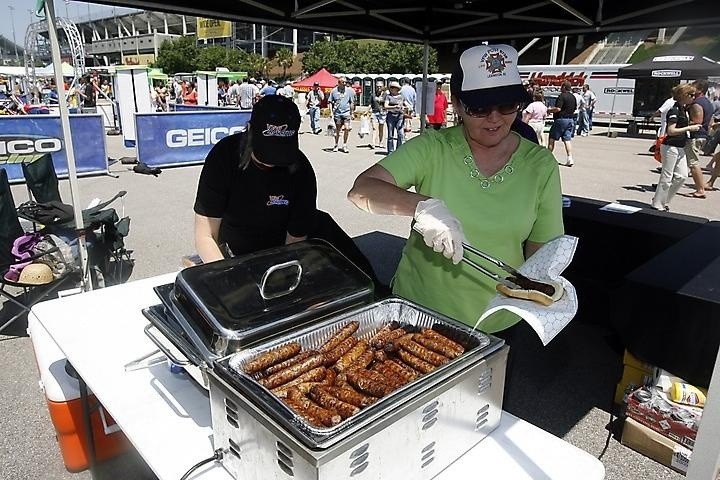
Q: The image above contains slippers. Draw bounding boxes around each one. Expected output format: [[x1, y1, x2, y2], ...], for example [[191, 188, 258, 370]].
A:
[[685, 192, 708, 198]]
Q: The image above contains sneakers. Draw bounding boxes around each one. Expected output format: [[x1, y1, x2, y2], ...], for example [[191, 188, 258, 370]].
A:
[[368, 139, 385, 149], [563, 160, 574, 167], [314, 128, 322, 135], [332, 144, 350, 152]]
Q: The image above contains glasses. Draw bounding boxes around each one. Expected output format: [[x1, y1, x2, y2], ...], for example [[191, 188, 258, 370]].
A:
[[689, 93, 696, 97], [456, 100, 523, 118]]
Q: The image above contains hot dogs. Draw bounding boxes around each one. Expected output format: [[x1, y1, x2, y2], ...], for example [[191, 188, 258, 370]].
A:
[[497, 275, 564, 307]]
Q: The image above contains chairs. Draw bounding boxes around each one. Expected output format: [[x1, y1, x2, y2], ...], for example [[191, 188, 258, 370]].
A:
[[0, 152, 131, 332]]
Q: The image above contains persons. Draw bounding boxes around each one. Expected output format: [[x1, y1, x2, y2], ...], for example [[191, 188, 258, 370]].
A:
[[649, 79, 720, 213], [518, 79, 597, 165], [347, 43, 565, 408], [194, 93, 317, 264]]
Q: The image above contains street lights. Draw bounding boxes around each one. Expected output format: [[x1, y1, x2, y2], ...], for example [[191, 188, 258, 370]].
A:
[[8, 5, 20, 63], [65, 0, 70, 18], [27, 8, 35, 24]]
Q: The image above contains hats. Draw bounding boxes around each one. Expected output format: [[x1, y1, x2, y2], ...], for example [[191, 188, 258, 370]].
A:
[[389, 81, 401, 90], [250, 94, 302, 166], [313, 81, 319, 86], [285, 80, 291, 85], [450, 43, 531, 104]]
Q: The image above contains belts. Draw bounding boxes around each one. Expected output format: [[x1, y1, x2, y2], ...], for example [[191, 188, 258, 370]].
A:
[[555, 115, 574, 119]]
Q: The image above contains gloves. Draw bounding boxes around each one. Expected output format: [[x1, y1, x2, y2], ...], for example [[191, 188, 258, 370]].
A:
[[413, 198, 471, 266]]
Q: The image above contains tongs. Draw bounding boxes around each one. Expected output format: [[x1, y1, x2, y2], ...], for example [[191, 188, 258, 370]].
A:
[[410, 221, 529, 290]]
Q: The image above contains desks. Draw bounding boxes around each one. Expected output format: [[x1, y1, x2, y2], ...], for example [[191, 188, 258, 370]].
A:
[[31, 271, 607, 480]]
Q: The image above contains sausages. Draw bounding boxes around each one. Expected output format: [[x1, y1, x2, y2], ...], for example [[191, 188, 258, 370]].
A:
[[242, 319, 466, 427]]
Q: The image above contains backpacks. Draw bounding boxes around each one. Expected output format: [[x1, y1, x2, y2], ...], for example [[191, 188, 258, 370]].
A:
[[34, 233, 75, 281]]
